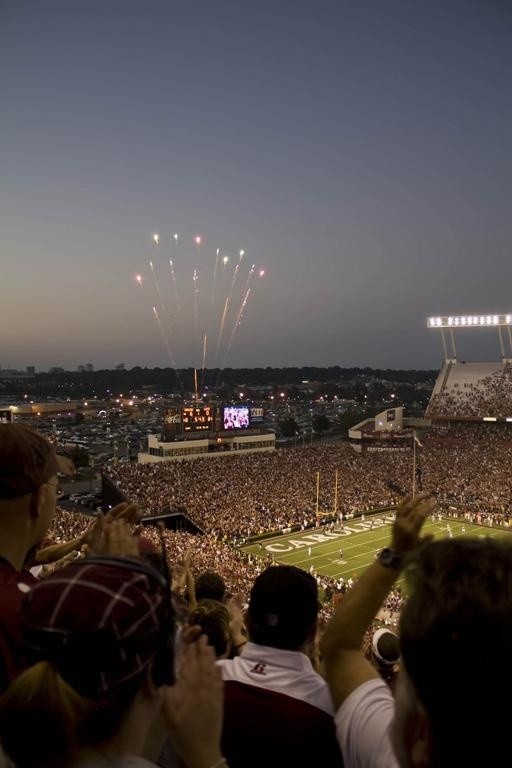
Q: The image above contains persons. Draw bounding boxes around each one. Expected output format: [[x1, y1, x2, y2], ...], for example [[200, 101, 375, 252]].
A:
[[103, 361, 512, 541], [0, 503, 512, 767], [1, 422, 143, 694]]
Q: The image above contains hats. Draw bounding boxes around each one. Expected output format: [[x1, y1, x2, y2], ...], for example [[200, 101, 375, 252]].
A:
[[0, 422, 63, 500], [23, 554, 166, 699], [372, 628, 401, 665], [251, 565, 323, 617]]
